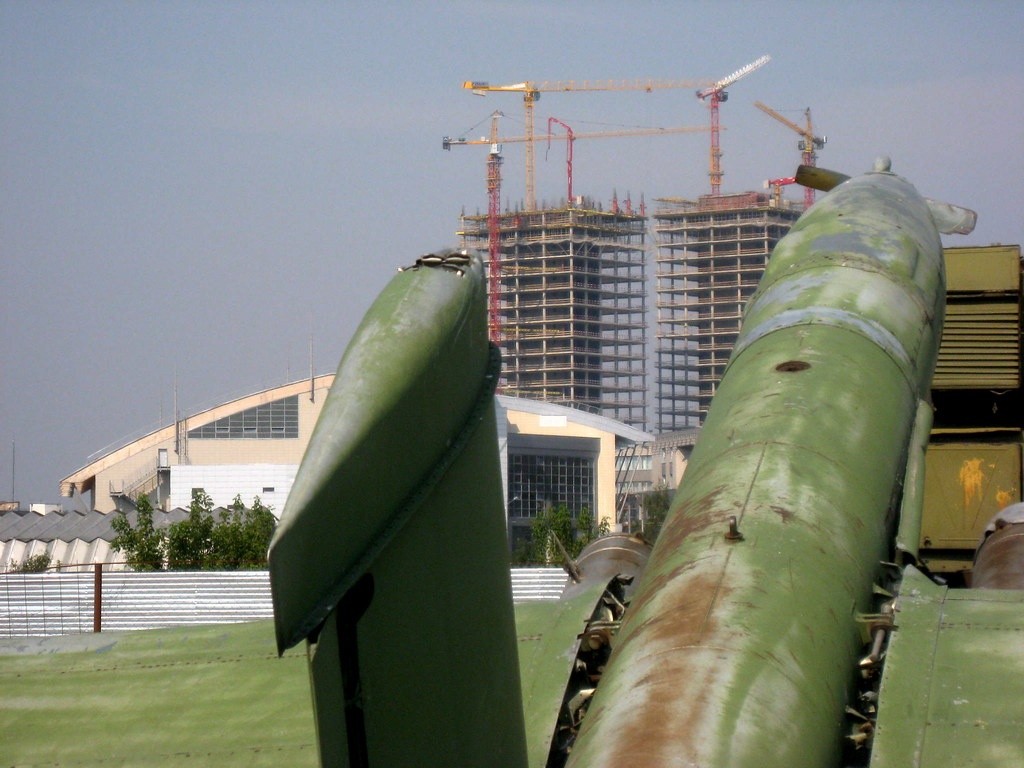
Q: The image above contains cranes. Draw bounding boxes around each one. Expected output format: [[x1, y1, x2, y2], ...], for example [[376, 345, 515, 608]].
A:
[[438, 54, 828, 207]]
